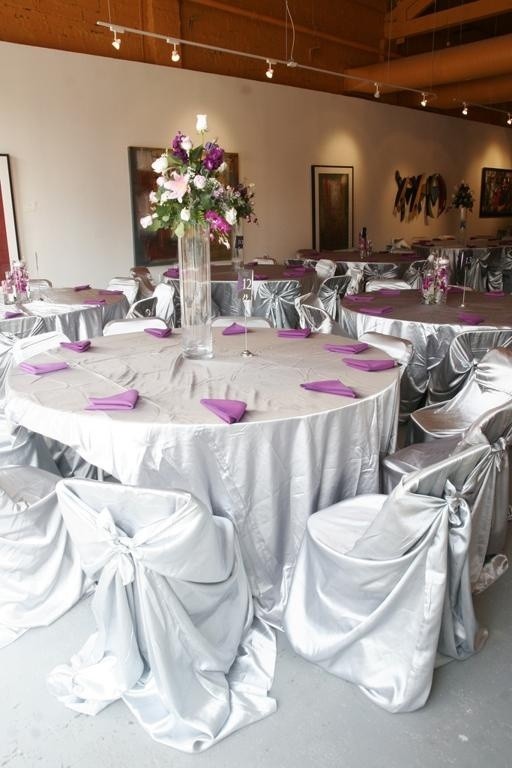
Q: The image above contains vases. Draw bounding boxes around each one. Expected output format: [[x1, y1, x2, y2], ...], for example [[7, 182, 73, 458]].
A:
[[457, 204, 468, 230], [176, 217, 217, 361]]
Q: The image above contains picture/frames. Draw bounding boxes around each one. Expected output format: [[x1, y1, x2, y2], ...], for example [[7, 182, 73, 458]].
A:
[[479, 165, 512, 219], [0, 153, 22, 286], [308, 162, 359, 255], [128, 145, 240, 271]]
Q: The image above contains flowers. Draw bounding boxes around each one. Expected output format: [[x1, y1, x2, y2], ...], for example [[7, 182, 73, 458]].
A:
[[450, 180, 475, 210], [140, 113, 260, 252]]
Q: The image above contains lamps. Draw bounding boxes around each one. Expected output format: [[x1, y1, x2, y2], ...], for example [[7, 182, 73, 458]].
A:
[[96, 20, 512, 124]]
